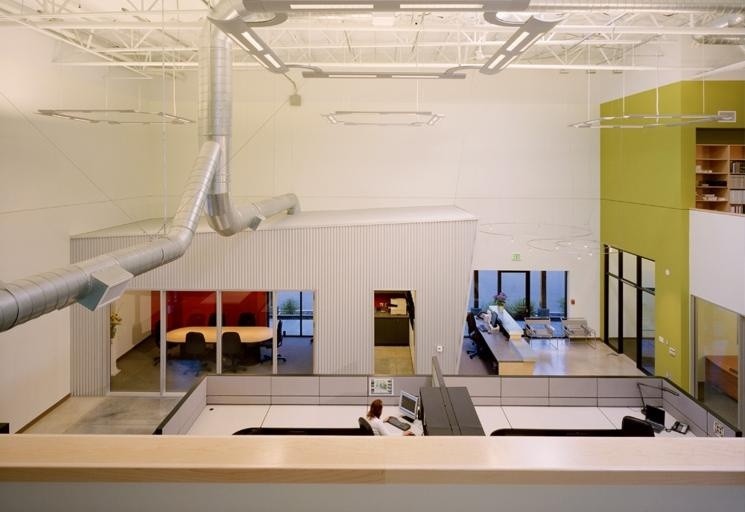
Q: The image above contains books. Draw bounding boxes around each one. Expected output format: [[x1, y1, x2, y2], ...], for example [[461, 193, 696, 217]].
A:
[[730, 160, 745, 213]]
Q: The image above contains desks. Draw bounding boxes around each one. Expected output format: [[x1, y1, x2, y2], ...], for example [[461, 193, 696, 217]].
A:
[[473, 404, 698, 437], [184, 404, 423, 438], [473, 315, 537, 375]]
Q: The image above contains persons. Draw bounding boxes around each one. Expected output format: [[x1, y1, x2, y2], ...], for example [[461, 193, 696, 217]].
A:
[[367, 399, 413, 436]]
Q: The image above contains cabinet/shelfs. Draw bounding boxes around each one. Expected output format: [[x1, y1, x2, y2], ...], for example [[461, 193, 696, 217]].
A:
[[697, 142, 745, 215]]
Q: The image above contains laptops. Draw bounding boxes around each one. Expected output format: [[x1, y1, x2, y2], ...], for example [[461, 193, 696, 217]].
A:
[[645, 405, 665, 433]]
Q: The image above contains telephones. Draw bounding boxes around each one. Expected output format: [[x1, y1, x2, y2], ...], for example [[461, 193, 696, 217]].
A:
[[671, 421, 689, 434]]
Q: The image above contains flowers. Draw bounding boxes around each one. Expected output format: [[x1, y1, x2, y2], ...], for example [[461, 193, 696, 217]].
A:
[[495, 292, 506, 304], [110, 312, 122, 327]]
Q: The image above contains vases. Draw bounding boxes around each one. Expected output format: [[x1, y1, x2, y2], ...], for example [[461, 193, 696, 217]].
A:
[[110, 325, 116, 339]]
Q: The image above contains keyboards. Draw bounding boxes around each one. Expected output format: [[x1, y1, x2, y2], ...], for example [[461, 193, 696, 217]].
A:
[[386, 416, 410, 431], [479, 325, 488, 332]]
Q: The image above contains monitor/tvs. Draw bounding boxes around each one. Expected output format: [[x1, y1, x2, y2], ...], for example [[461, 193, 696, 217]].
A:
[[400, 391, 417, 423], [491, 311, 498, 327]]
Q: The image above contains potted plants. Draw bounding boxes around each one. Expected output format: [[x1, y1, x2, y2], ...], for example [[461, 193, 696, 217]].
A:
[[498, 305, 504, 313]]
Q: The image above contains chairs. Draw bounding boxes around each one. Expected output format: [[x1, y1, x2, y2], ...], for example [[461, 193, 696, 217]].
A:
[[466, 313, 484, 359], [524, 316, 596, 351], [153, 313, 287, 376]]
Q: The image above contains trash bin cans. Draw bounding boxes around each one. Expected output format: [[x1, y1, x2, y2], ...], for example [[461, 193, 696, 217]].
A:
[[698, 381, 705, 400]]
